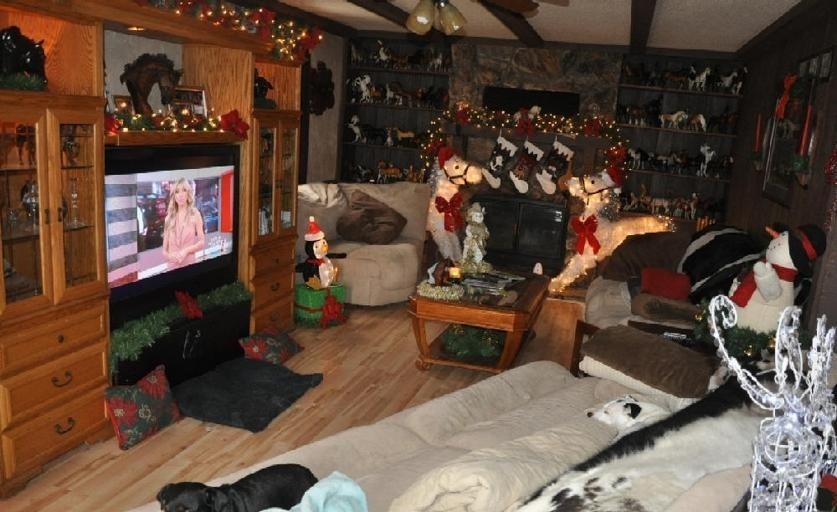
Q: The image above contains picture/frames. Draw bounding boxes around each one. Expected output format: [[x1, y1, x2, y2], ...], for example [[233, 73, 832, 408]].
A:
[[112, 94, 136, 119], [166, 85, 208, 118], [797, 45, 834, 82]]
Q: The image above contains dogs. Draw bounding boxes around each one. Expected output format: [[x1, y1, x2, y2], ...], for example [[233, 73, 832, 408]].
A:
[[156, 463, 318, 512], [587, 394, 671, 444]]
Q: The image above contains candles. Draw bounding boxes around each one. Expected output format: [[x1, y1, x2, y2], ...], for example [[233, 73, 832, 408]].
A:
[[798, 104, 813, 158], [752, 112, 762, 151]]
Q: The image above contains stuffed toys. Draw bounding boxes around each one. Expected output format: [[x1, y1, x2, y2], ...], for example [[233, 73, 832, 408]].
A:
[[725, 219, 826, 337], [460, 200, 489, 263], [294, 213, 348, 327]]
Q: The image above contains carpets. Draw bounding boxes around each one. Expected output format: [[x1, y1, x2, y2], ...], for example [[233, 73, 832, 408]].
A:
[[174, 358, 322, 433]]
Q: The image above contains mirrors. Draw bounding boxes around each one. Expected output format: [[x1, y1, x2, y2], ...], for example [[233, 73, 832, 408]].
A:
[[761, 76, 817, 206]]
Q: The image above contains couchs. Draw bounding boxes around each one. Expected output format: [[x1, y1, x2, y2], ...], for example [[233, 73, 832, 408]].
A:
[[134, 325, 809, 511]]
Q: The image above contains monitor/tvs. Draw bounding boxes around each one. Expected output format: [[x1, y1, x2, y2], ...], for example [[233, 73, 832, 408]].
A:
[[105, 145, 241, 330]]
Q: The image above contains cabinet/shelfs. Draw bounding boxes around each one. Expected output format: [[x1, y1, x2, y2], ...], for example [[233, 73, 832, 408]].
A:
[[1, 91, 109, 487], [470, 194, 566, 276], [614, 83, 743, 224], [245, 109, 296, 341], [345, 61, 449, 155]]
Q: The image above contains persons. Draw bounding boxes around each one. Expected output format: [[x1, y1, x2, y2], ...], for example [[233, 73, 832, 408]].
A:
[[160, 177, 207, 273]]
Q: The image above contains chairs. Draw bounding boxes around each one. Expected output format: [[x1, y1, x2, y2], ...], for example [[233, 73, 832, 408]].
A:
[[298, 183, 433, 306], [570, 226, 809, 376]]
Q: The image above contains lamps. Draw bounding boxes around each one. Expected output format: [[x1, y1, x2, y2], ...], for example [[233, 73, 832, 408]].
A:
[[406, 0, 468, 36]]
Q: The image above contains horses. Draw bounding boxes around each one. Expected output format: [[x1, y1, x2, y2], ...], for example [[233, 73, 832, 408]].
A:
[[513, 105, 541, 124], [121, 53, 184, 114]]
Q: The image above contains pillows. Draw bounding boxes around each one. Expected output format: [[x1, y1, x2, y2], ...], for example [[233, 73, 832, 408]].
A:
[[640, 267, 691, 301], [105, 368, 179, 449], [338, 190, 408, 246], [235, 325, 304, 364]]
